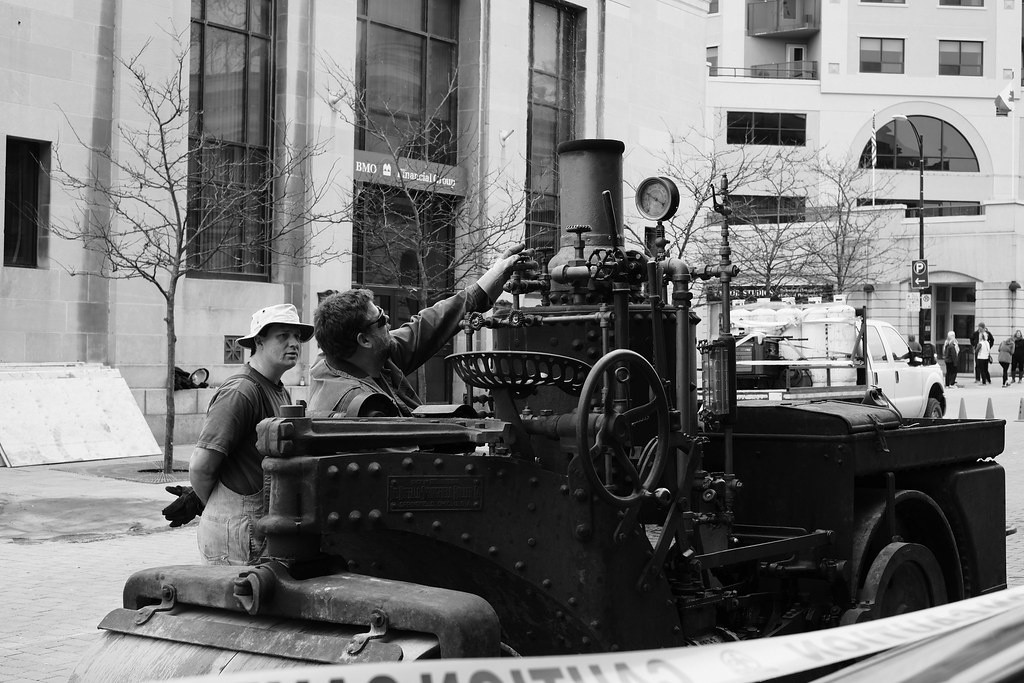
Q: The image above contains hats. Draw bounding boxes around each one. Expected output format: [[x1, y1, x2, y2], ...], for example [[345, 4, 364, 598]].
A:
[[236, 304, 315, 349]]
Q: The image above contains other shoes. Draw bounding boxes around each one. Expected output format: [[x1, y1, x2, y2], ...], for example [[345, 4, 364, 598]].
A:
[[1018, 380, 1022, 383], [945, 384, 958, 389], [974, 380, 992, 386], [1002, 383, 1010, 388], [1011, 380, 1015, 383]]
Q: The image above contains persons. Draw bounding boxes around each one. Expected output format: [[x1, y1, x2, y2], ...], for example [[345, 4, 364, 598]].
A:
[[163, 303, 315, 566], [907, 322, 1024, 389], [304, 244, 526, 422]]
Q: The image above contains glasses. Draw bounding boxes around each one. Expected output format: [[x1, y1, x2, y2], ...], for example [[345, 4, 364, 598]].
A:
[[371, 306, 389, 328]]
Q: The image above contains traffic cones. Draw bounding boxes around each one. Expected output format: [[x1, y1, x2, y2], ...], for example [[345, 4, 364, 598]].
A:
[[985, 397, 995, 419], [958, 397, 968, 419], [1013, 397, 1024, 422]]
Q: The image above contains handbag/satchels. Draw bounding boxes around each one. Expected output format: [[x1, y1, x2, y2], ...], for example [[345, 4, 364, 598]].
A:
[[989, 356, 993, 364]]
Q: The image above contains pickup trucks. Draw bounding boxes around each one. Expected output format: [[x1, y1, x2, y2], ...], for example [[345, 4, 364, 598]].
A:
[[724, 299, 948, 418]]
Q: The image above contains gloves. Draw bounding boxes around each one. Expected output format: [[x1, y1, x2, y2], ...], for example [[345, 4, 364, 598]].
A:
[[162, 485, 205, 527], [476, 243, 539, 302]]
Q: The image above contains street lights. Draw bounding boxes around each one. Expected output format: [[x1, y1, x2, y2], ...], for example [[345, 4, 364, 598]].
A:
[[893, 114, 925, 346]]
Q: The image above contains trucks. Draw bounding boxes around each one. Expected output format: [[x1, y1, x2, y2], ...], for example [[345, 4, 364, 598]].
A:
[[691, 297, 949, 420]]
